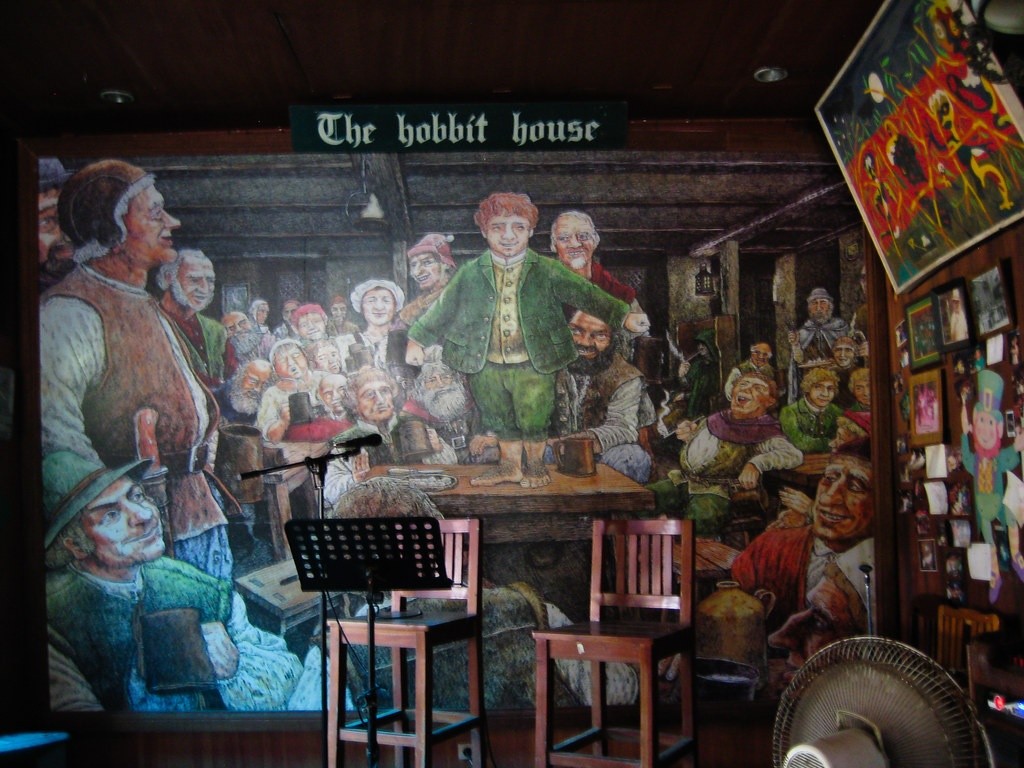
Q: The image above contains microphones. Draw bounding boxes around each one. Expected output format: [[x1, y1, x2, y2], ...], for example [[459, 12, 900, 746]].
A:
[[333, 433, 382, 449]]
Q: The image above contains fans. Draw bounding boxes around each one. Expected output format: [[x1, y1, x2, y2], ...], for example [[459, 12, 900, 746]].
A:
[[770, 635, 993, 768]]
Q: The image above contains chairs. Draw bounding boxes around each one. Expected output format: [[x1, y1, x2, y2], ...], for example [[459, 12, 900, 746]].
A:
[[320, 518, 487, 768], [530, 517, 698, 768]]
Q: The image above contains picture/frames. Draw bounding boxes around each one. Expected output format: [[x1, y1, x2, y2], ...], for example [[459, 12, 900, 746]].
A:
[[902, 288, 949, 373], [931, 276, 977, 356], [967, 255, 1017, 344], [908, 367, 946, 448], [813, 0, 1024, 298]]
